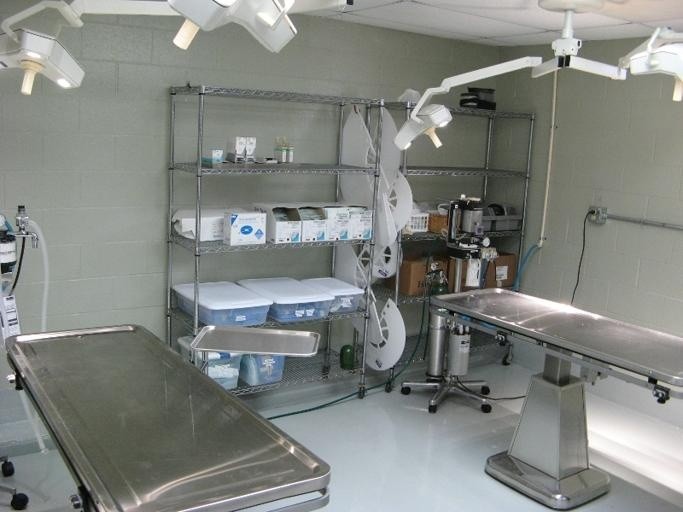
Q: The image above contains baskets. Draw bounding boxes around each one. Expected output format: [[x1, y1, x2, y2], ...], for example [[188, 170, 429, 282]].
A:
[[408, 213, 429, 233], [428, 214, 448, 233]]
